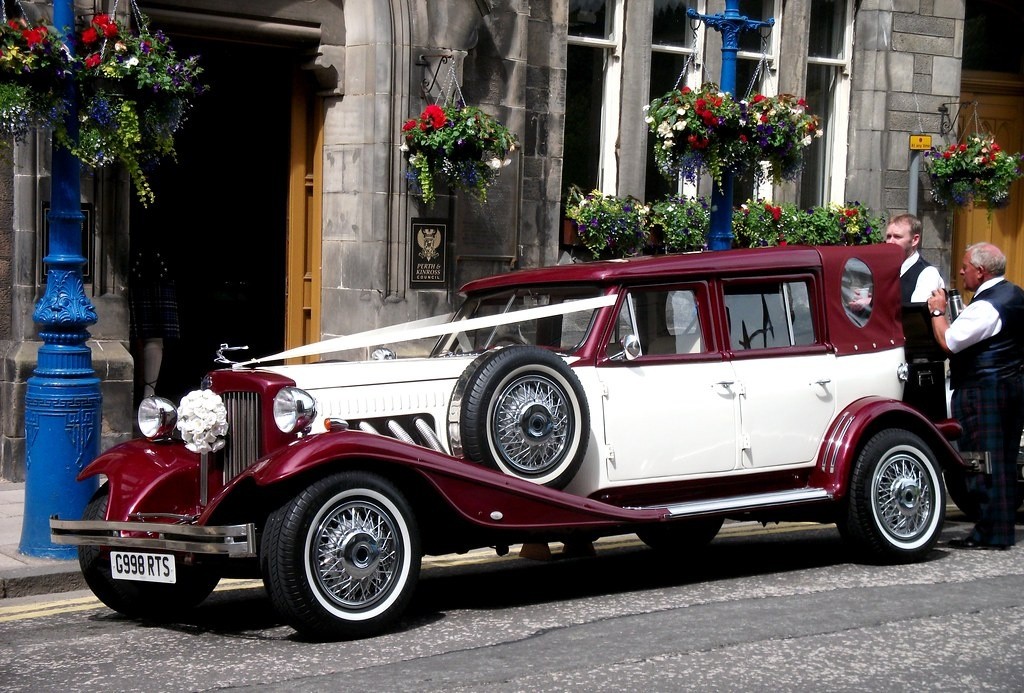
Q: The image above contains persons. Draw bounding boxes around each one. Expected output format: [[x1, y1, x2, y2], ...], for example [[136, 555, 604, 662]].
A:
[[927, 243, 1024, 551], [848, 214, 948, 423]]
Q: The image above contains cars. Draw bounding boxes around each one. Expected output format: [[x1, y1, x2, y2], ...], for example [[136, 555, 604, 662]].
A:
[[48, 244, 993, 640]]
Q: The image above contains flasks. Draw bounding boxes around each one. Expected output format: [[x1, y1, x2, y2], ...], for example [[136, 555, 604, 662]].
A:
[[948, 289, 964, 324]]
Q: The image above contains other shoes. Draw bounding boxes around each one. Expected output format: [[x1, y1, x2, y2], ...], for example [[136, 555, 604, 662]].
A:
[[563, 540, 596, 558], [520, 543, 552, 561]]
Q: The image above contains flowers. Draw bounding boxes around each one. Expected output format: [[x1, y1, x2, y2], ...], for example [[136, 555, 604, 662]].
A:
[[924, 142, 972, 177], [176, 389, 229, 455], [77, 8, 212, 97], [967, 131, 1024, 186], [643, 81, 749, 197], [402, 102, 519, 209], [730, 197, 891, 249], [0, 11, 61, 75], [736, 90, 823, 195], [561, 183, 711, 260]]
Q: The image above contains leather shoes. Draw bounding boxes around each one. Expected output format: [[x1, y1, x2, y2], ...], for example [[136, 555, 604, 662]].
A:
[[947, 535, 1011, 550]]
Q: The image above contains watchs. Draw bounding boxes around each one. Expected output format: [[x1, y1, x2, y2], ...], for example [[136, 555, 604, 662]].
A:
[[931, 309, 945, 318]]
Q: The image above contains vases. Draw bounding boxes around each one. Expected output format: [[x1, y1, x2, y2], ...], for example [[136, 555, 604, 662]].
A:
[[980, 168, 1010, 209], [564, 220, 664, 247], [0, 53, 54, 94], [406, 135, 482, 170], [930, 167, 971, 207], [81, 74, 132, 104]]
